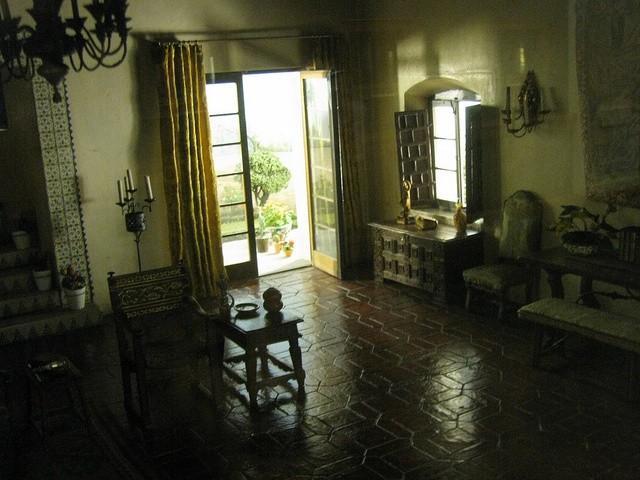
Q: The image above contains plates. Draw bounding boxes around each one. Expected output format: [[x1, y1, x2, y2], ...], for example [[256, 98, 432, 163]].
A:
[[233, 302, 260, 314]]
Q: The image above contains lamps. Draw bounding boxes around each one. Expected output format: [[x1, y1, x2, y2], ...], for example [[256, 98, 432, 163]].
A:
[[1, 1, 131, 106], [500, 69, 557, 140]]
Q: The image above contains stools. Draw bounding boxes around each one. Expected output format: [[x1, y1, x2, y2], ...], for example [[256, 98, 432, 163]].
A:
[[21, 347, 88, 441]]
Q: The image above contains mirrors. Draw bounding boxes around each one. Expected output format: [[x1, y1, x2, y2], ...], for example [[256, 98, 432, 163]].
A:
[[430, 90, 478, 216]]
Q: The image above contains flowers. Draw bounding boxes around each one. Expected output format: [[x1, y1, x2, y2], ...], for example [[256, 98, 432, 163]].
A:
[[58, 264, 89, 290]]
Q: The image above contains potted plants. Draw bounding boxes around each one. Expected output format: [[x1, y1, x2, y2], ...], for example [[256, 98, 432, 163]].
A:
[[31, 265, 55, 295], [272, 231, 284, 255], [10, 222, 34, 252], [283, 241, 296, 257], [235, 146, 293, 254], [546, 197, 626, 260]]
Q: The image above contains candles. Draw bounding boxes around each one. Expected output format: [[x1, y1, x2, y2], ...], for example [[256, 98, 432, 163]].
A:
[[127, 170, 137, 194], [522, 94, 528, 125], [117, 179, 126, 207], [506, 95, 511, 120], [506, 86, 510, 111], [143, 172, 157, 202], [124, 177, 132, 203], [541, 87, 545, 113]]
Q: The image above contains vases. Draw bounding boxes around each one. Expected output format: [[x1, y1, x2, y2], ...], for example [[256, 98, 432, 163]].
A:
[[58, 287, 88, 313]]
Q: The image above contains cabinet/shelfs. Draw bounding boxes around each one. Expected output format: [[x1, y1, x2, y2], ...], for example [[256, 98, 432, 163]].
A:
[[365, 216, 484, 297]]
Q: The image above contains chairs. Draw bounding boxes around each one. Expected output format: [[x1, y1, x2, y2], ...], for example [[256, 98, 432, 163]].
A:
[[109, 263, 219, 437], [463, 187, 544, 321]]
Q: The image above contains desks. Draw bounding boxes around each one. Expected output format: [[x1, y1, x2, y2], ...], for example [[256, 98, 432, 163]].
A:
[[208, 295, 308, 410], [544, 239, 639, 309]]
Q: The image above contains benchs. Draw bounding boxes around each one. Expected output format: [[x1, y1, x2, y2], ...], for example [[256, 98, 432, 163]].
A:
[[517, 297, 639, 376]]
